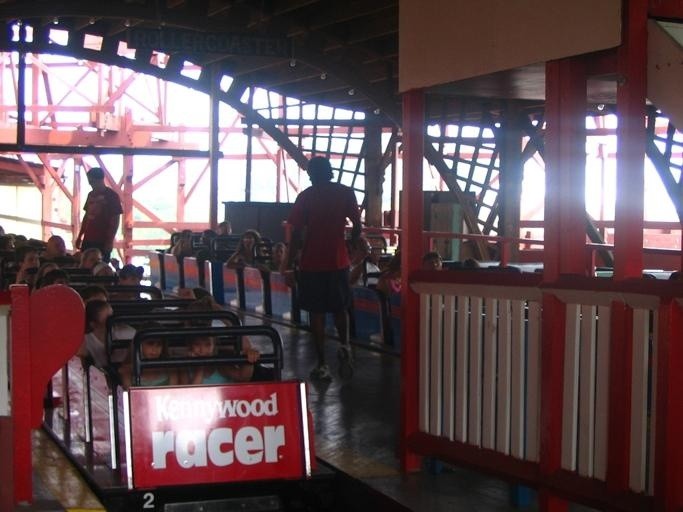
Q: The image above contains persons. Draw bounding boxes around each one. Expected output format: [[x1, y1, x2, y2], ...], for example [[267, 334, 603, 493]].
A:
[[277, 155, 364, 388], [344, 218, 482, 304], [74, 168, 124, 258], [164, 221, 287, 273], [1, 226, 261, 392]]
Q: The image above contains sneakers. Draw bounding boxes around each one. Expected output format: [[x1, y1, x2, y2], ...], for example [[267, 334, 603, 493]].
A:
[[309, 363, 333, 382], [336, 344, 353, 381]]
